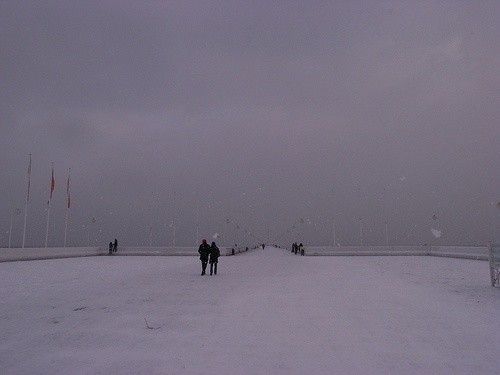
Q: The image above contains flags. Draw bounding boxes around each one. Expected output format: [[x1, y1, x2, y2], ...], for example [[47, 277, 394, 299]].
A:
[[27, 162, 31, 199], [48, 171, 55, 204], [66, 178, 70, 208]]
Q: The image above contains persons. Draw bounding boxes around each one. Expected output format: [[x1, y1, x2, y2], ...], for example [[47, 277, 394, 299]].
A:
[[113, 239, 118, 252], [198, 240, 210, 276], [262, 244, 265, 250], [209, 242, 220, 276], [109, 242, 113, 256], [291, 242, 303, 254]]
